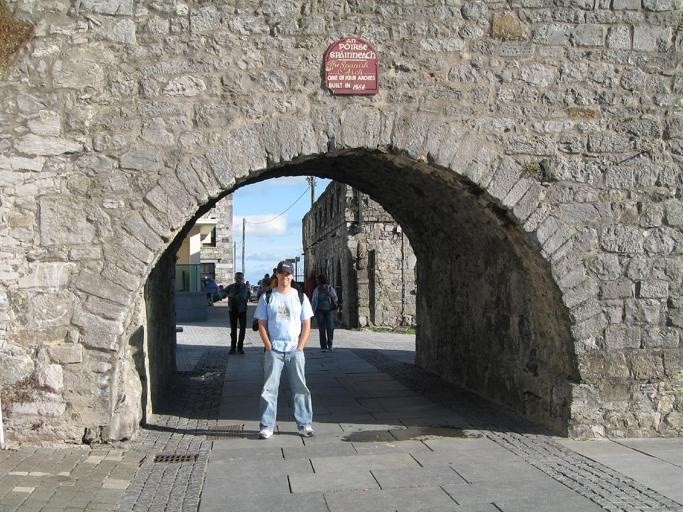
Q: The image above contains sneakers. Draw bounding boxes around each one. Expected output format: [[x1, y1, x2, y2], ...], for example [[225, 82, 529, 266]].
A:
[[327, 342, 332, 351], [229, 348, 236, 354], [297, 425, 315, 437], [257, 426, 274, 439], [237, 348, 244, 354]]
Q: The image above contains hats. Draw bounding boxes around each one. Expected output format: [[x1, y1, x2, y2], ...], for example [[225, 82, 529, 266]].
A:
[[277, 261, 294, 275]]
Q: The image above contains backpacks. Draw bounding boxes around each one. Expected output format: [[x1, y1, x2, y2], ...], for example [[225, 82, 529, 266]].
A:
[[317, 284, 337, 312]]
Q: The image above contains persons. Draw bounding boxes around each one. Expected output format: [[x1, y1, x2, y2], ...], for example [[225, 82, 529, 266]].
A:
[[220, 272, 248, 354], [254, 261, 315, 439], [246, 268, 314, 303], [311, 274, 338, 352]]
